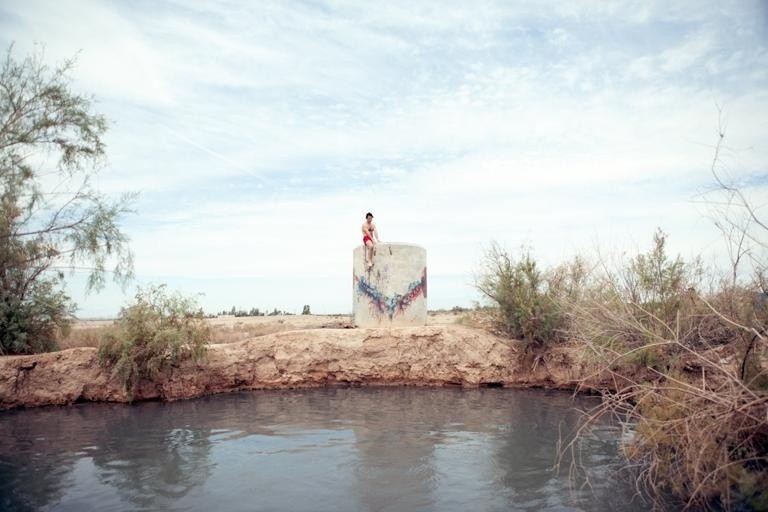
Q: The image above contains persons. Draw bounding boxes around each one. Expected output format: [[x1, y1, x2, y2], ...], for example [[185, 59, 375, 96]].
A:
[[362, 212, 380, 267]]
